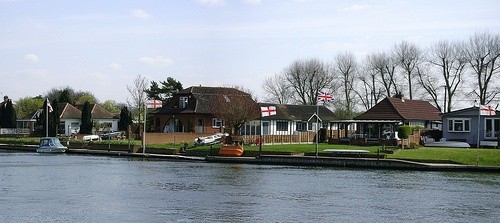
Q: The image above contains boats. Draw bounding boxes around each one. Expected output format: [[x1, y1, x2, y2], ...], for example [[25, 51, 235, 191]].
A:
[[218, 140, 244, 157], [83, 131, 124, 141], [194, 132, 229, 147], [35, 136, 68, 153]]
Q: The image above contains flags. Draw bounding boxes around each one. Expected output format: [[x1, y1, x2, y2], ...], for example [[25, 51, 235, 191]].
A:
[[47, 99, 53, 112], [261, 106, 277, 117], [318, 91, 335, 103], [147, 100, 162, 108], [480, 105, 496, 116]]
